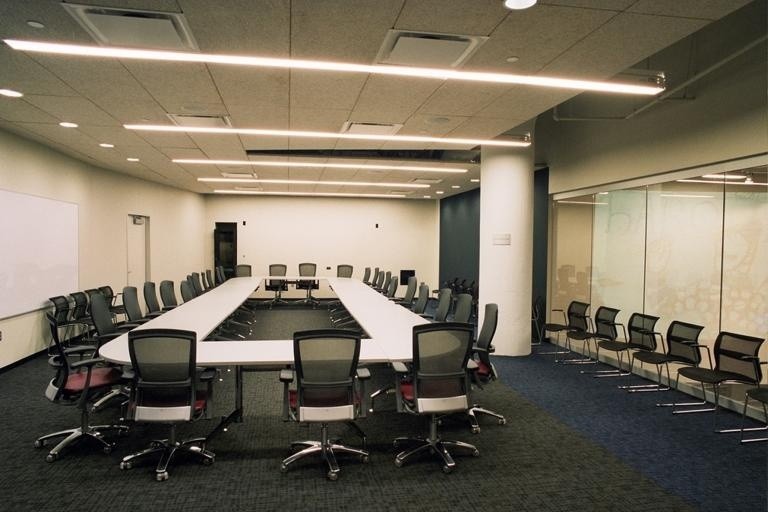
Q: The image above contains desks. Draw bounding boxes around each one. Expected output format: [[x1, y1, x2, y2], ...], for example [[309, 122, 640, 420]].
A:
[[99, 277, 462, 450]]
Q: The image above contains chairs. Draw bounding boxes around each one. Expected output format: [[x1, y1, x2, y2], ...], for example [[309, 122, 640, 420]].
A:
[[293, 262, 320, 307], [526, 292, 768, 449], [276, 327, 371, 481], [391, 320, 480, 471], [235, 264, 252, 277], [49, 266, 227, 411], [362, 266, 506, 433], [263, 263, 289, 310], [336, 264, 353, 278], [117, 328, 216, 481], [35, 310, 130, 461]]
